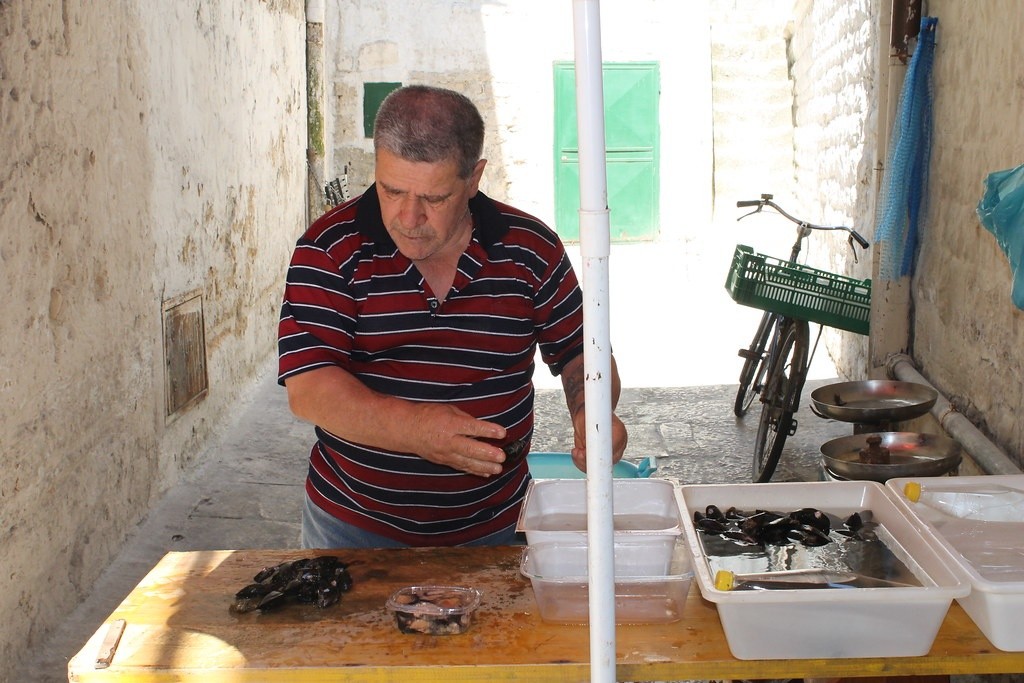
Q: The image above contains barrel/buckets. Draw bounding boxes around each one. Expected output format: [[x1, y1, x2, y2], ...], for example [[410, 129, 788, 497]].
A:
[[525, 452, 657, 478]]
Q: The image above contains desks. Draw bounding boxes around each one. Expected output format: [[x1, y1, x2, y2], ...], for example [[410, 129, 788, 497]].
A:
[[67, 547, 1024, 683]]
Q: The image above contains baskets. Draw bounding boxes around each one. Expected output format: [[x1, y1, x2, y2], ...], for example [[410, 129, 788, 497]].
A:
[[725, 244, 872, 336]]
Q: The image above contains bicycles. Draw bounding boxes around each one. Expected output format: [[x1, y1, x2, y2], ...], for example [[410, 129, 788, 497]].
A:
[[725, 193, 873, 484]]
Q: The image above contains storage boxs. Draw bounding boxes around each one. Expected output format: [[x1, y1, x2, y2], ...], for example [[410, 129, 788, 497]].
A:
[[724, 244, 872, 336], [385, 585, 484, 636], [885, 474, 1024, 652], [674, 479, 972, 661], [518, 542, 694, 624], [514, 476, 683, 583]]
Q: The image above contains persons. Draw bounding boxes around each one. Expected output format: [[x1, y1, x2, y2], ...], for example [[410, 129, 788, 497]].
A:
[[278, 86, 627, 550]]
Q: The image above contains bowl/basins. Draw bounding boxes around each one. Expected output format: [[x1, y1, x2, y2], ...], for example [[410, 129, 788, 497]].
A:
[[384, 585, 486, 635]]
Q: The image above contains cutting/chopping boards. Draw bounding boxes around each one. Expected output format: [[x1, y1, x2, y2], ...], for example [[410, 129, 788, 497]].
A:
[[68, 545, 1024, 683]]
